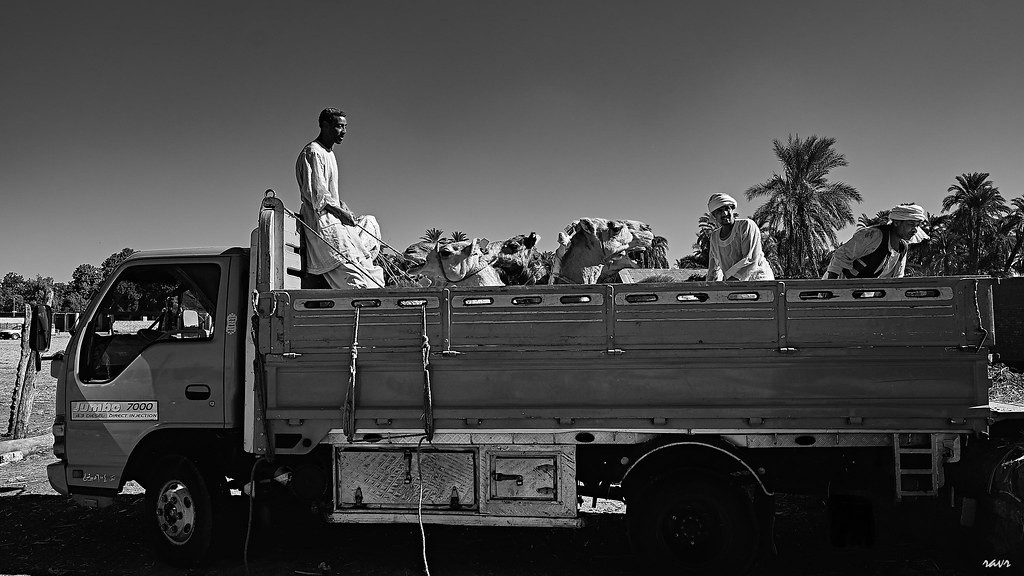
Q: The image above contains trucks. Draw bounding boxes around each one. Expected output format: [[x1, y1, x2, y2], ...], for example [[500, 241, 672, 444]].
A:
[[29, 188, 997, 576]]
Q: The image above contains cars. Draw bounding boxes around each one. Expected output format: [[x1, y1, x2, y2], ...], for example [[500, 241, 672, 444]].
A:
[[0, 325, 22, 340]]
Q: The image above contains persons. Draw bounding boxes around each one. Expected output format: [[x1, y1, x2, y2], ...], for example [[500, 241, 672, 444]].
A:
[[294, 106, 389, 309], [817, 204, 926, 298], [703, 192, 774, 300]]
